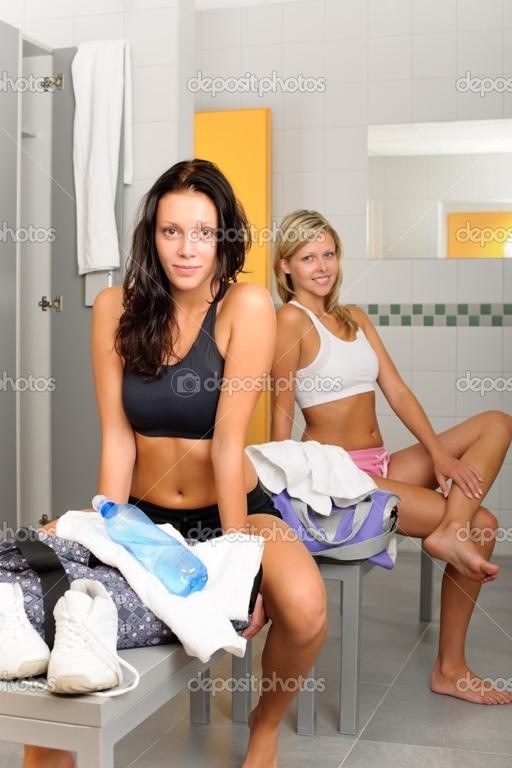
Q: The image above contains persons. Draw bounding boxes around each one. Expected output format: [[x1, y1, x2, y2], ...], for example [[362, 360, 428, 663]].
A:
[[262, 207, 511, 706], [37, 157, 321, 767]]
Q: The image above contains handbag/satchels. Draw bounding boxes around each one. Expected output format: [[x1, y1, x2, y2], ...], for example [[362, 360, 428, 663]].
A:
[[258, 480, 399, 569], [0, 527, 263, 647]]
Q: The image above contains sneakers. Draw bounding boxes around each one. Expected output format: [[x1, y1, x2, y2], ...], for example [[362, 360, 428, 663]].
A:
[[47, 579, 118, 695], [1, 582, 50, 681]]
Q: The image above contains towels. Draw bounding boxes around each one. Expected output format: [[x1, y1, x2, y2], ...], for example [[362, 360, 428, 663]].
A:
[[70, 37, 134, 278], [54, 509, 267, 665], [244, 438, 380, 517]]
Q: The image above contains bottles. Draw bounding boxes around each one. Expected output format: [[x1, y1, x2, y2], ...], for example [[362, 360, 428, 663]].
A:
[[91, 493, 208, 596]]
